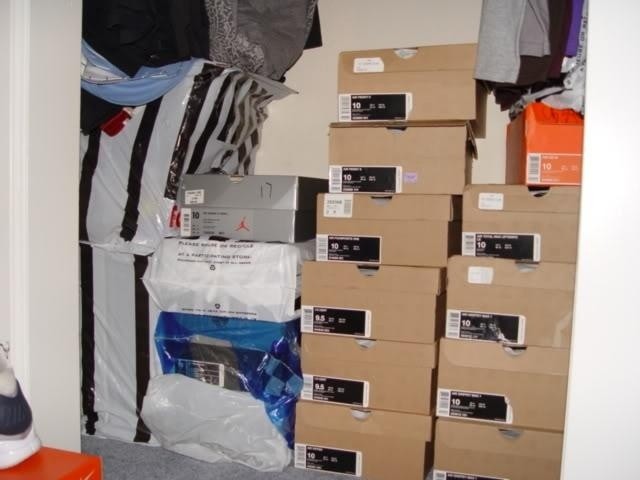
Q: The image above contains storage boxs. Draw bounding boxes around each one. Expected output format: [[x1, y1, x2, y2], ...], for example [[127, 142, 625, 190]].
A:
[[173, 41, 583, 478]]
